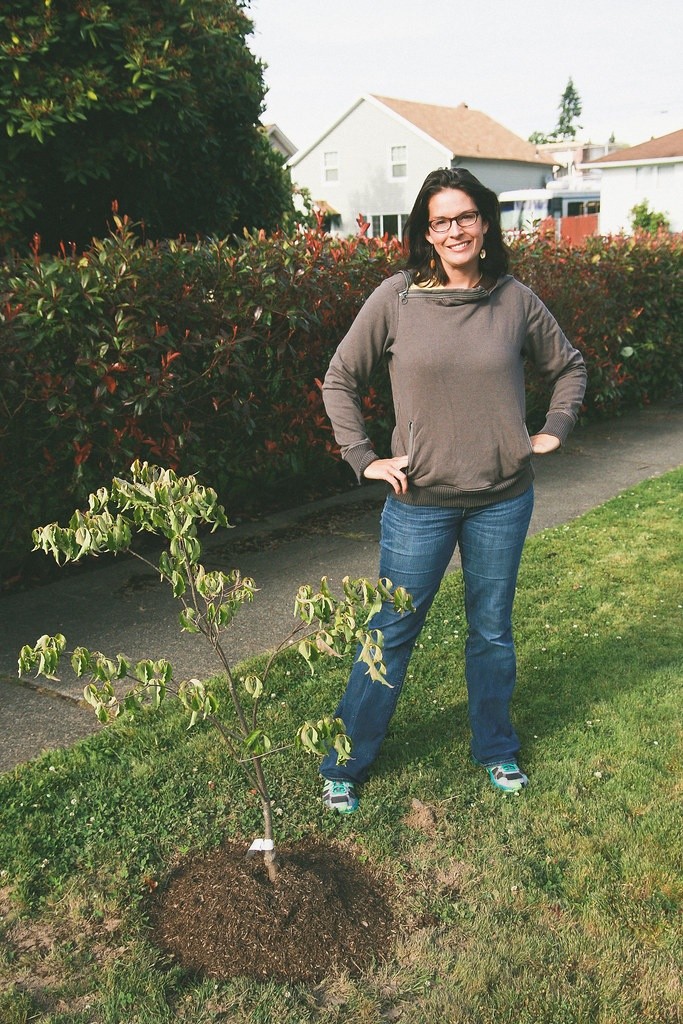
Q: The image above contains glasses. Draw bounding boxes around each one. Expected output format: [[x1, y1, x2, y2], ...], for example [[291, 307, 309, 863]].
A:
[[427, 210, 481, 232]]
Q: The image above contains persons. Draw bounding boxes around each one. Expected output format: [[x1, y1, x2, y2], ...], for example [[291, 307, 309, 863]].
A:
[[321, 166, 586, 814]]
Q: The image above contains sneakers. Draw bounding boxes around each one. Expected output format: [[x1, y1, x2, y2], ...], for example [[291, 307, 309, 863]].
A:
[[472, 753, 529, 794], [322, 775, 358, 814]]
